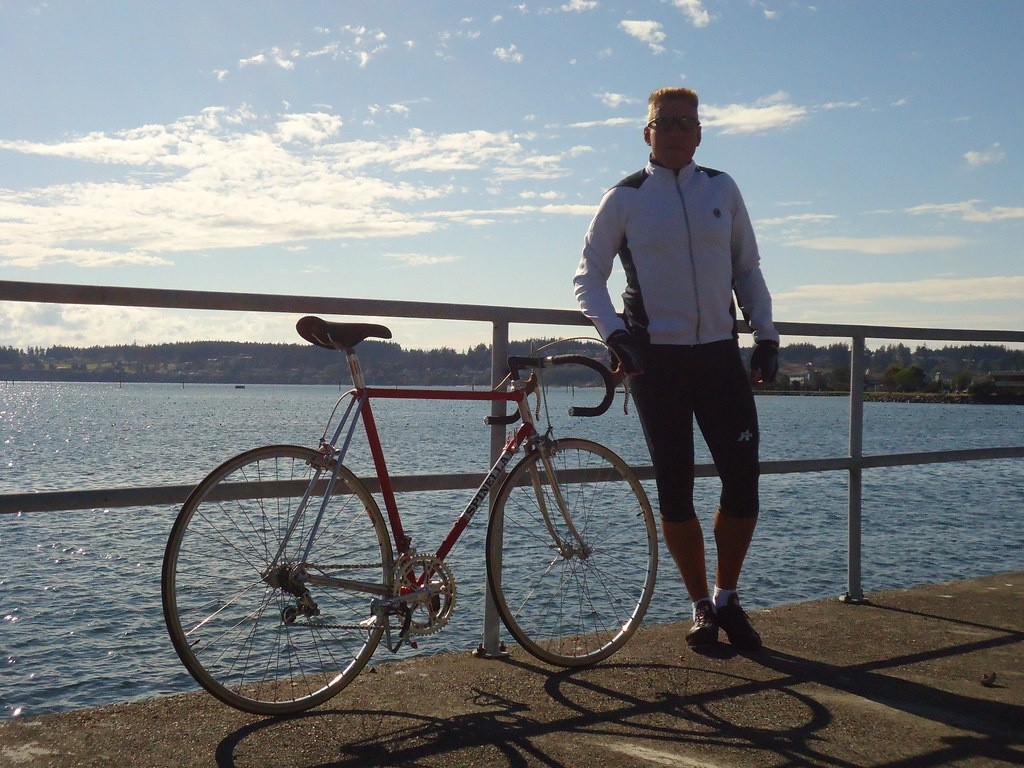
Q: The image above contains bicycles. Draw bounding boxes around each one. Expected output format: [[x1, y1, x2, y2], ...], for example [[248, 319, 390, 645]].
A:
[[160, 314, 659, 715]]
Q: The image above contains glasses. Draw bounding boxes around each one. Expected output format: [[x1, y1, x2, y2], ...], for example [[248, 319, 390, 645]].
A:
[[647, 115, 701, 132]]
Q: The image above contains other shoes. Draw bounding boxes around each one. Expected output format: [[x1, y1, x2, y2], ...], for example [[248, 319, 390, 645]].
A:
[[712, 590, 760, 647], [685, 600, 719, 648]]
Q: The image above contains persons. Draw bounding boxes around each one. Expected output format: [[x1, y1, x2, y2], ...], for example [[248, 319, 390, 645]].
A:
[[574, 89, 781, 648]]
